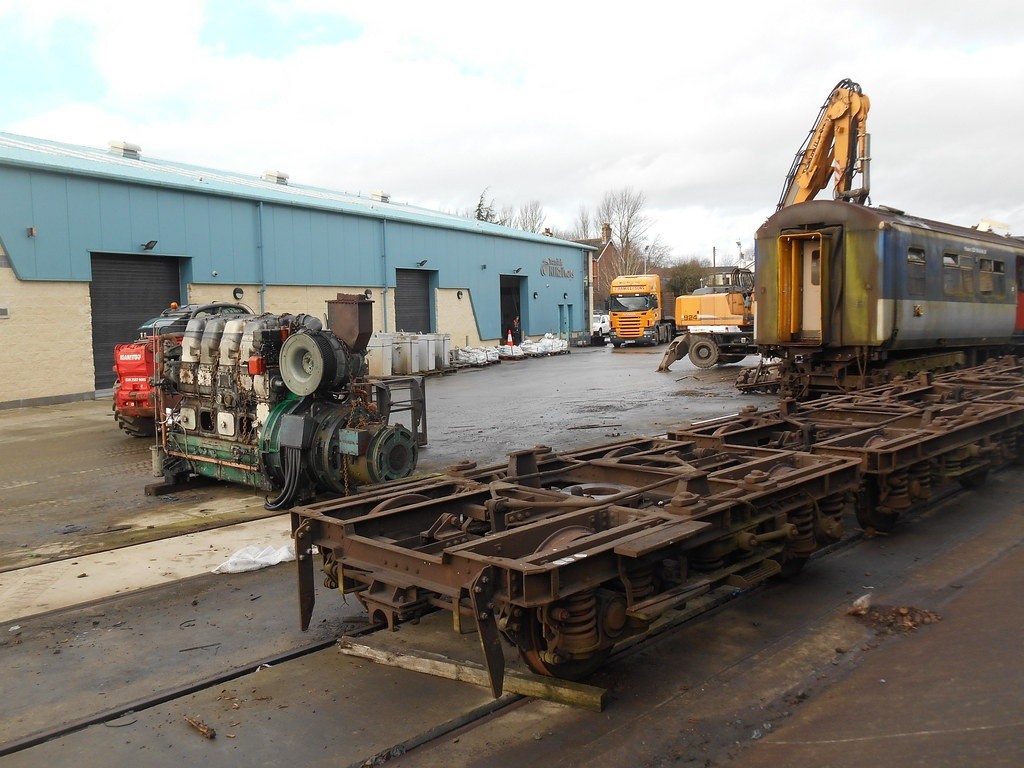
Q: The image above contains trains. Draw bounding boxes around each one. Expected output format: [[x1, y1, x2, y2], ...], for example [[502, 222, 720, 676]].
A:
[[752, 201, 1024, 399]]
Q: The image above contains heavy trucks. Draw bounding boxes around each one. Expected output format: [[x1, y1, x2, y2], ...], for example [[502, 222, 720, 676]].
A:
[[604, 273, 676, 348]]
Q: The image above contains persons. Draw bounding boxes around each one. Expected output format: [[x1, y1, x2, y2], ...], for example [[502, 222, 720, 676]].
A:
[[511, 315, 521, 345]]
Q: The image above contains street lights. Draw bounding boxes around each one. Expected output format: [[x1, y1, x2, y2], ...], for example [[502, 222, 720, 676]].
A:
[[644, 245, 650, 275]]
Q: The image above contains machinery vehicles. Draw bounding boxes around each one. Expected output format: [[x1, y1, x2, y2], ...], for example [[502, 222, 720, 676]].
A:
[[656, 78, 874, 374]]
